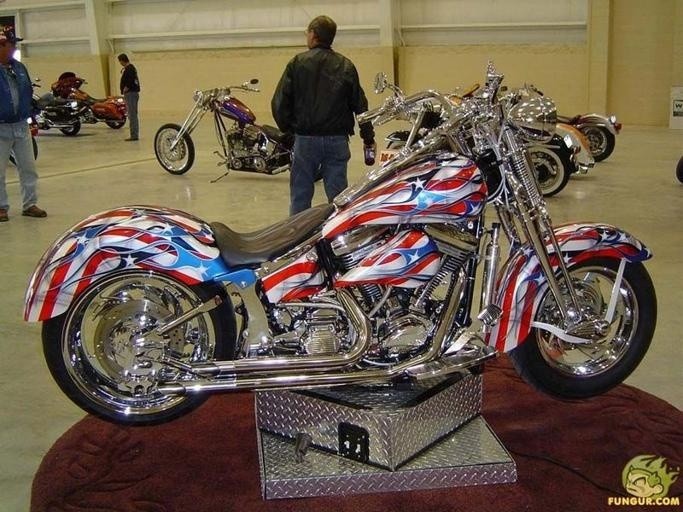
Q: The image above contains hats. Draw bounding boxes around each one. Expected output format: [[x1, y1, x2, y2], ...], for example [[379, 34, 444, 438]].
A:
[[1, 26, 24, 42]]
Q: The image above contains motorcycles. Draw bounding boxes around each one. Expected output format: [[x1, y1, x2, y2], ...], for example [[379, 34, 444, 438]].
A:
[[9, 100, 38, 165], [31, 78, 81, 136], [50, 72, 128, 129], [153, 79, 324, 183], [384, 82, 621, 199], [23, 59, 658, 426]]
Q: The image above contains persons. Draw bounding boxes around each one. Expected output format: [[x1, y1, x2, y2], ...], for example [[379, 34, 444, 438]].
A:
[[117, 53, 138, 140], [271, 14, 376, 218], [0, 24, 46, 222]]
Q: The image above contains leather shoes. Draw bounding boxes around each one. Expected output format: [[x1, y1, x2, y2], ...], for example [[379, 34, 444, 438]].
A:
[[22, 205, 47, 218], [0, 209, 9, 222], [125, 138, 138, 141]]
